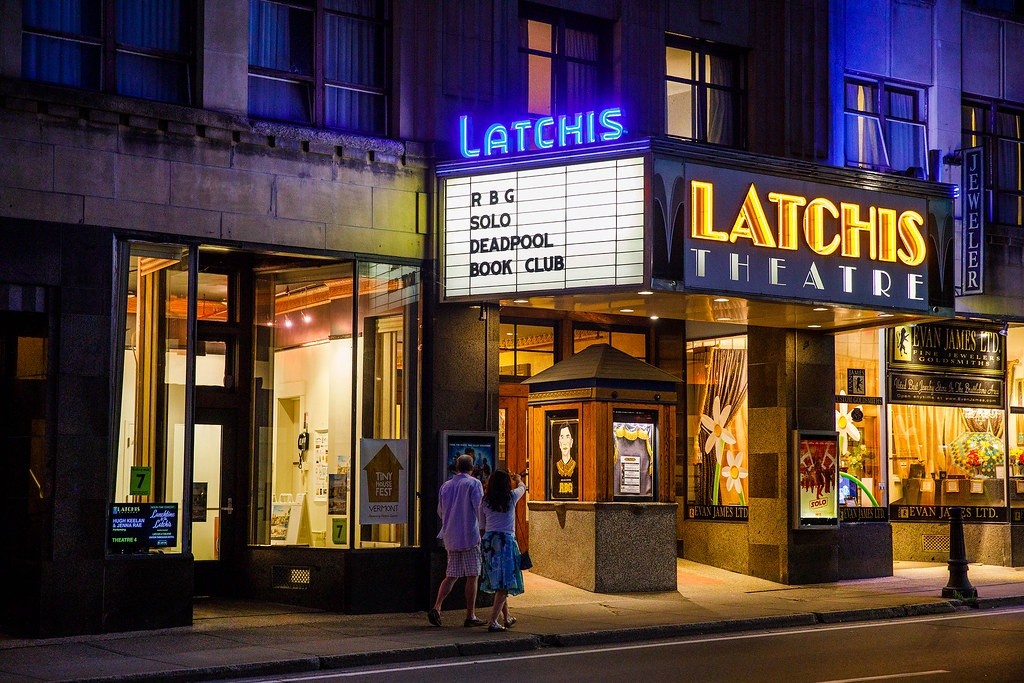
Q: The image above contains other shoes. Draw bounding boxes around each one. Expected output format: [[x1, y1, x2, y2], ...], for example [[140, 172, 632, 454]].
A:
[[503, 617, 516, 627], [488, 622, 508, 631]]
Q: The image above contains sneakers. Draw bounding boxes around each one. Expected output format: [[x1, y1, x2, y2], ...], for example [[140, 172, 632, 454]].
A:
[[464, 616, 486, 626], [428, 609, 442, 626]]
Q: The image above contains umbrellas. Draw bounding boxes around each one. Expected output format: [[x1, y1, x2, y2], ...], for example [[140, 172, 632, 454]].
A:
[[948, 431, 1006, 479]]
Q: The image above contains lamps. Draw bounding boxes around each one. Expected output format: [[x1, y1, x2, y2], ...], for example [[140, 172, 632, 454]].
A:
[[284, 310, 311, 327]]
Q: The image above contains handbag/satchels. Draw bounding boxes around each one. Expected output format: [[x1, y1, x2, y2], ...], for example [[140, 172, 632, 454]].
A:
[[521, 550, 532, 570]]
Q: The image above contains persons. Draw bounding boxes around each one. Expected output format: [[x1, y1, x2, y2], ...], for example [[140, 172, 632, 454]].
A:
[[447, 447, 491, 495], [428, 455, 488, 628], [479, 468, 526, 632], [801, 457, 835, 499]]
